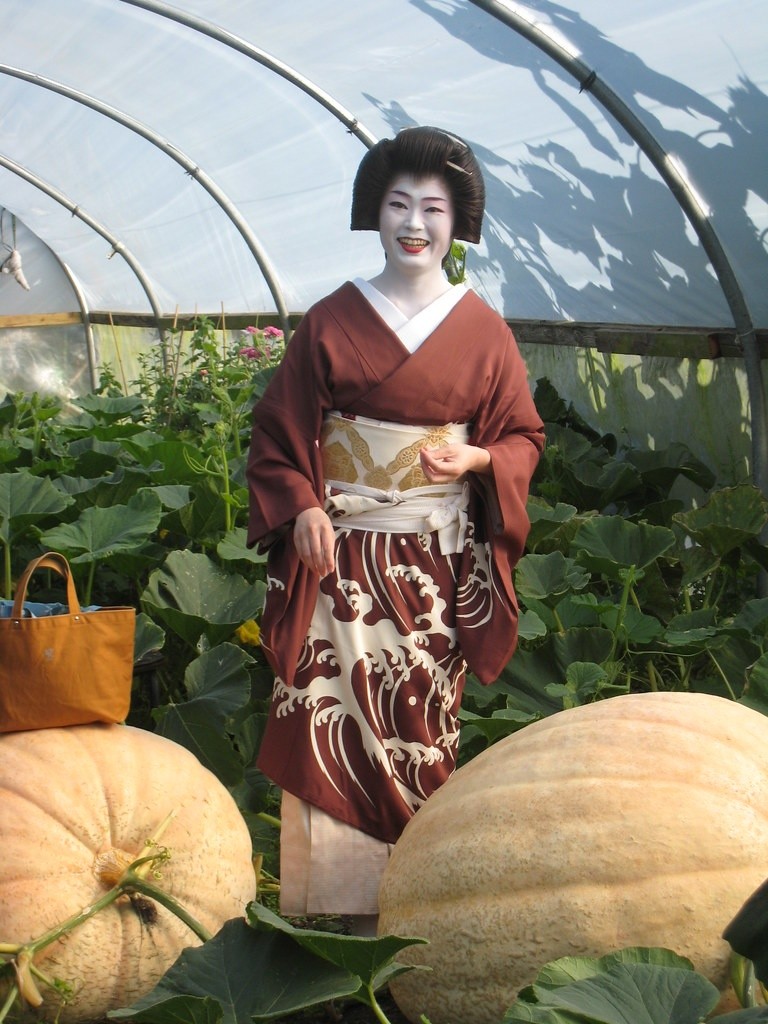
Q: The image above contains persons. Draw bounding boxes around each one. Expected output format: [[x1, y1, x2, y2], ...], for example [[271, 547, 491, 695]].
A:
[[245, 126, 544, 914]]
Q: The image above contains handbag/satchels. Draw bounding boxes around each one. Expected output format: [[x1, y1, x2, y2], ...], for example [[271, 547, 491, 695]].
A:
[[1, 551, 133, 739]]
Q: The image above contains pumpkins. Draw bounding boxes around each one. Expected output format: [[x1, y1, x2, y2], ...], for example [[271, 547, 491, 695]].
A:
[[376, 694, 768, 1024], [0, 726, 257, 1018]]
